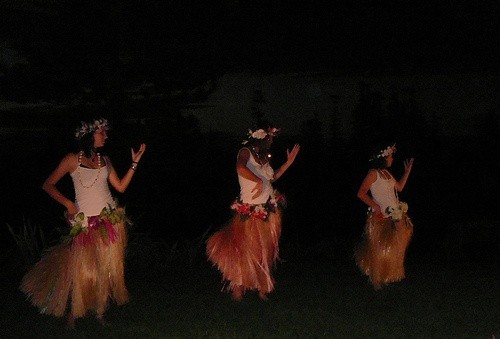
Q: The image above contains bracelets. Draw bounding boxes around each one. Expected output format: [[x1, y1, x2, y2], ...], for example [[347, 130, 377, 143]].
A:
[[130, 165, 137, 173], [132, 161, 138, 164]]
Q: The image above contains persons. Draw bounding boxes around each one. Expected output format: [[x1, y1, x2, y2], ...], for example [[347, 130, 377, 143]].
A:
[[17, 117, 146, 333], [205, 128, 300, 303], [354, 140, 414, 293]]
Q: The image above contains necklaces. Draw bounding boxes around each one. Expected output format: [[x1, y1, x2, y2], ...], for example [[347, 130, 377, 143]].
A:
[[249, 143, 274, 181], [379, 169, 398, 198], [77, 150, 101, 188]]
